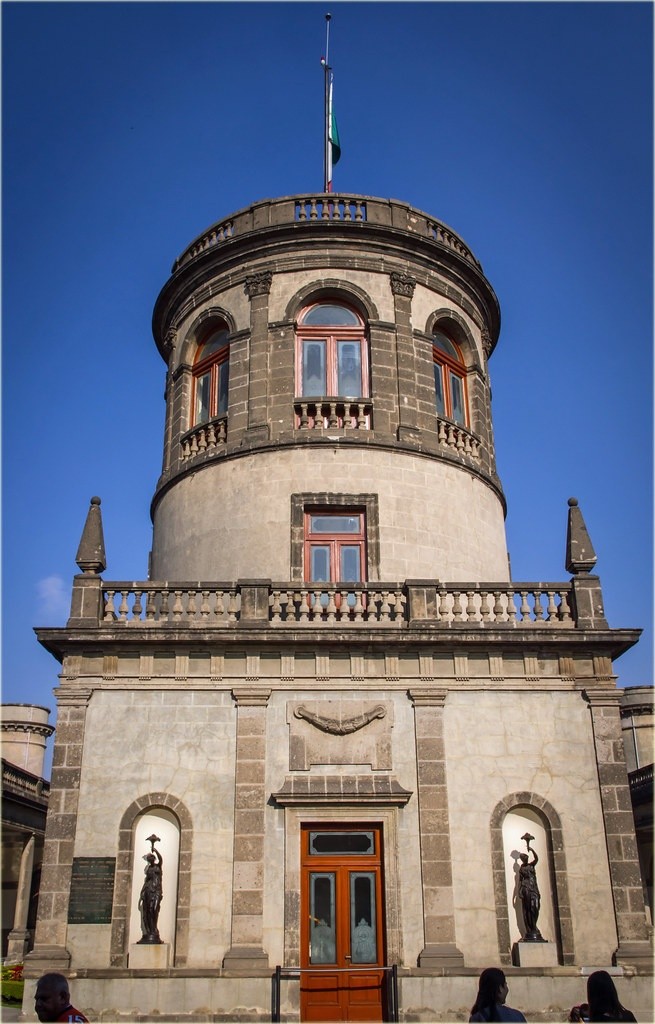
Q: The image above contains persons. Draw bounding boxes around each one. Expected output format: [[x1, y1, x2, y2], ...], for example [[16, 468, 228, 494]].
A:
[[34, 973, 89, 1024], [518, 847, 541, 935], [140, 849, 163, 935], [469, 967, 526, 1024], [564, 970, 637, 1024]]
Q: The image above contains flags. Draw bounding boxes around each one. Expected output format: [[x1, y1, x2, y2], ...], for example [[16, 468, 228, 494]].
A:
[[329, 90, 341, 164]]
